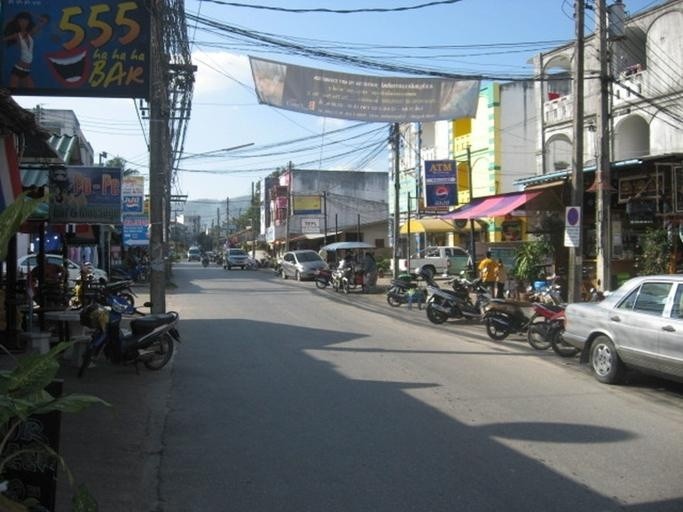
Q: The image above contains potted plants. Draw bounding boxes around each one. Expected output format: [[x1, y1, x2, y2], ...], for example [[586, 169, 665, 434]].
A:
[[511, 242, 543, 303]]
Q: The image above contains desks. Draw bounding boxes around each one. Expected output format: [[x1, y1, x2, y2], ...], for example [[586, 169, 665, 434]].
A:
[[43, 310, 81, 349]]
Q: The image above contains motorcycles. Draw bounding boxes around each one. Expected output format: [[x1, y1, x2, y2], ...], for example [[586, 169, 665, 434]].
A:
[[310, 266, 351, 294], [386, 274, 605, 357], [201, 255, 208, 267], [67, 277, 182, 377]]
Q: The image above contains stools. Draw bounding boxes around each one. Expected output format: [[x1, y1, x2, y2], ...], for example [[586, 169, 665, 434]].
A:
[[16, 331, 53, 355], [69, 335, 97, 369]]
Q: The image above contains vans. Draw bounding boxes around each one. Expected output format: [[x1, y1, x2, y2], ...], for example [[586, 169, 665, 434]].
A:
[[186, 246, 201, 261]]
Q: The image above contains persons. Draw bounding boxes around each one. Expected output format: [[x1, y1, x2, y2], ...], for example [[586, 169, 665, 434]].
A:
[[254, 62, 287, 107], [361, 252, 377, 289], [25, 252, 68, 333], [476, 251, 497, 300], [492, 257, 512, 299], [340, 250, 357, 270], [0, 11, 49, 91]]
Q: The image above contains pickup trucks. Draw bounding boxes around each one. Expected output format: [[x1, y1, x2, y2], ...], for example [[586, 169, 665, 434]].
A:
[[389, 245, 469, 279]]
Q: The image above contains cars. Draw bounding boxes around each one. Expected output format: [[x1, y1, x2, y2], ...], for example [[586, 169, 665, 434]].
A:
[[17, 254, 108, 283], [279, 250, 329, 282], [223, 248, 272, 270], [563, 274, 683, 384]]
